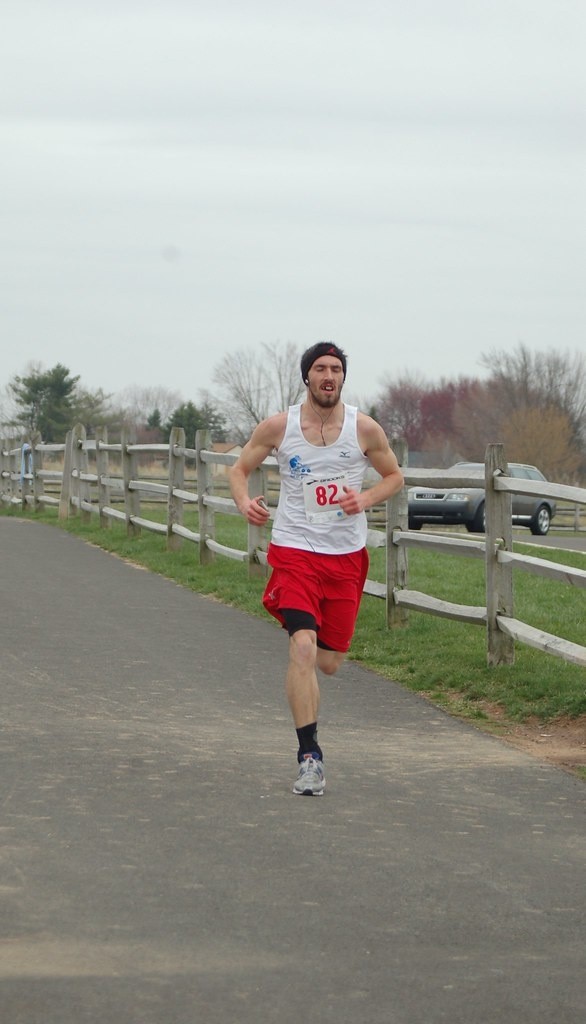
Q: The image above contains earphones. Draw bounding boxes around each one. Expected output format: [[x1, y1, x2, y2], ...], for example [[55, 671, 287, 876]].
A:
[[305, 379, 309, 384]]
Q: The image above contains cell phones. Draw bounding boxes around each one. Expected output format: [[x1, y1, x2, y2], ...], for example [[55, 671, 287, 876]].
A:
[[257, 500, 268, 512]]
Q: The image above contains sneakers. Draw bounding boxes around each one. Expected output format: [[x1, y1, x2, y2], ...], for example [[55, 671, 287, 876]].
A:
[[292, 752, 327, 795]]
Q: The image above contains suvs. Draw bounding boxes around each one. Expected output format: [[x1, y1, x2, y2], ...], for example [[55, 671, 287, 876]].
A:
[[406, 459, 558, 536]]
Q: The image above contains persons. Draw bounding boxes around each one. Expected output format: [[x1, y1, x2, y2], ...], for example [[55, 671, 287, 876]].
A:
[[228, 342, 405, 796]]
